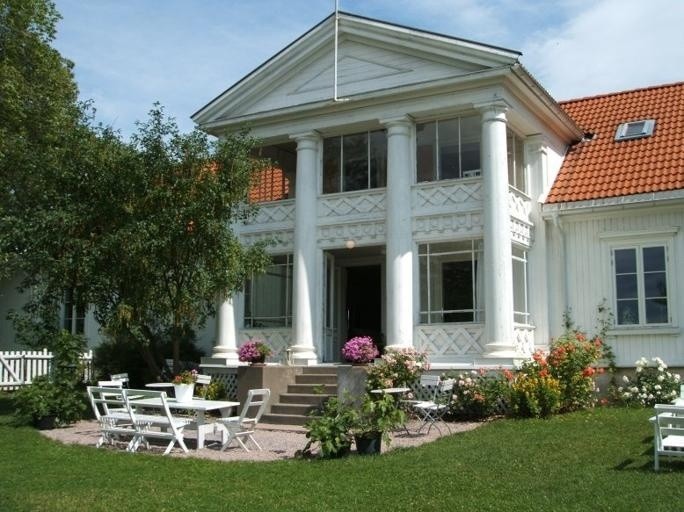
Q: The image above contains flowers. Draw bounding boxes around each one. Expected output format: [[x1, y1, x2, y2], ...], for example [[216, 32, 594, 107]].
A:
[[238, 339, 271, 362], [172, 368, 199, 385], [341, 335, 380, 364]]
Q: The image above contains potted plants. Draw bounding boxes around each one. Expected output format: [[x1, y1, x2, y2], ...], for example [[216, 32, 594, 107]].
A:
[[302, 384, 358, 459], [351, 389, 406, 456], [7, 374, 86, 432]]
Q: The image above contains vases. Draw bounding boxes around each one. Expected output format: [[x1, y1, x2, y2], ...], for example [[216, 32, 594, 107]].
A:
[[252, 353, 265, 363], [175, 383, 195, 402]]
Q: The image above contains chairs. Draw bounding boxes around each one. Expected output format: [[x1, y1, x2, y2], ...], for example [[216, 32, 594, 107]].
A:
[[216, 389, 270, 452], [399, 375, 440, 432], [191, 374, 212, 422], [110, 372, 145, 418], [412, 378, 456, 439], [97, 381, 145, 441], [649, 404, 684, 471]]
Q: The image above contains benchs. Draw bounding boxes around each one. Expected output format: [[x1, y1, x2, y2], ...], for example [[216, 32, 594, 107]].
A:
[[87, 386, 190, 456]]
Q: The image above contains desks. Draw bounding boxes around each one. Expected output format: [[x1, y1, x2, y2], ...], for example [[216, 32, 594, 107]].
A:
[[370, 386, 412, 435], [145, 383, 177, 416], [125, 396, 240, 449]]
